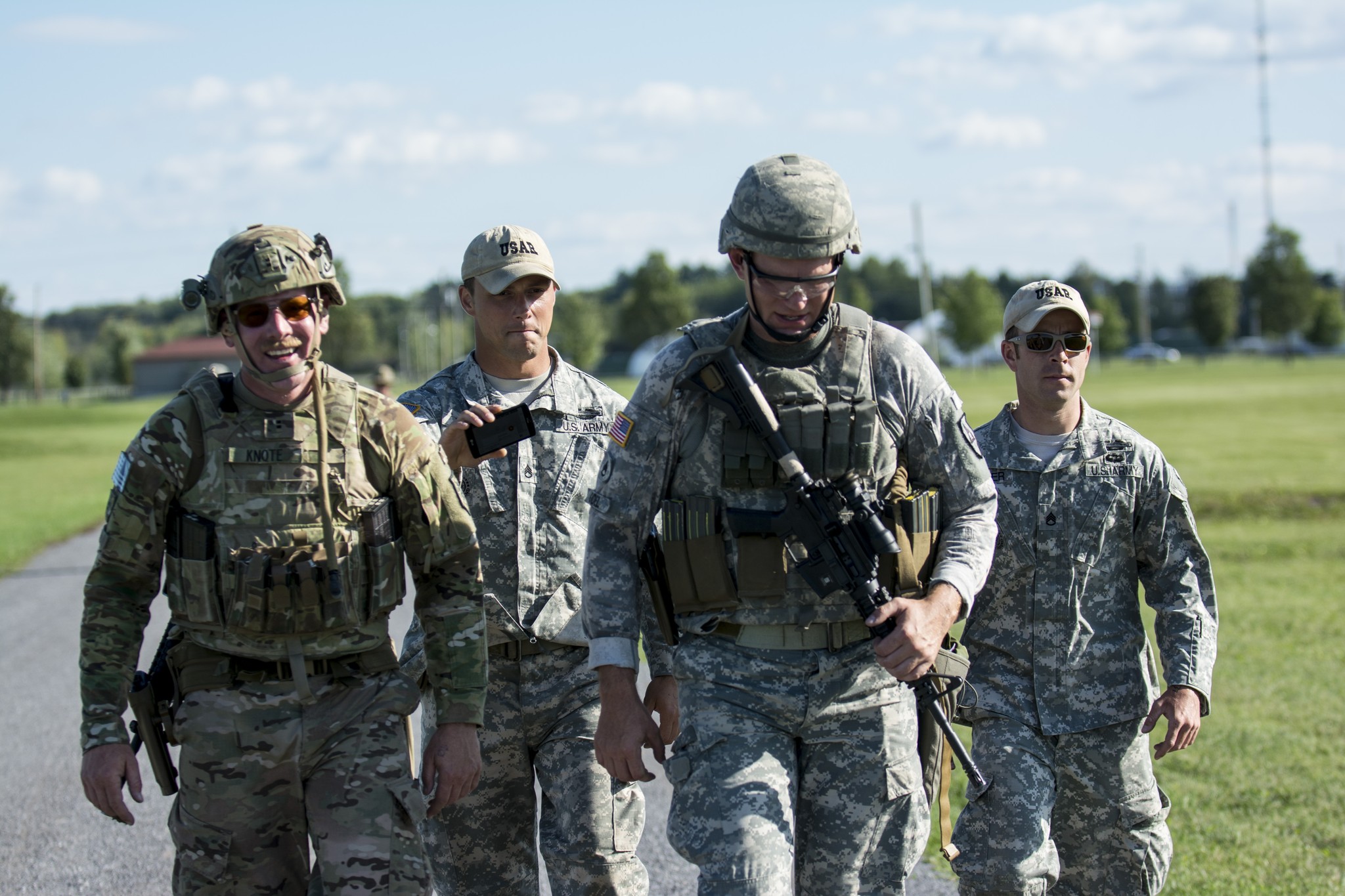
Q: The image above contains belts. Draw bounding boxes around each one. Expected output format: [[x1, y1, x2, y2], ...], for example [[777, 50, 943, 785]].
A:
[[487, 637, 569, 660], [711, 618, 885, 654], [180, 638, 399, 693]]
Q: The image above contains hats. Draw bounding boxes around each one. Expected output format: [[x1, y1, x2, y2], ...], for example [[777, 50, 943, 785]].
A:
[[1002, 280, 1090, 337], [461, 225, 560, 295]]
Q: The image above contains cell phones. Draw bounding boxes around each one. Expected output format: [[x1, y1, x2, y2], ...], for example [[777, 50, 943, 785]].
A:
[[466, 403, 536, 459]]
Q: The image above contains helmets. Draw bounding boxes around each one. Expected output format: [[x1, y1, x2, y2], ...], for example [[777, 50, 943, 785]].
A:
[[718, 153, 865, 260], [203, 224, 347, 338]]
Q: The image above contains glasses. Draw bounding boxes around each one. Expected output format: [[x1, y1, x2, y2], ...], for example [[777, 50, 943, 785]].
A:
[[1007, 333, 1091, 352], [224, 296, 318, 327], [744, 256, 840, 299]]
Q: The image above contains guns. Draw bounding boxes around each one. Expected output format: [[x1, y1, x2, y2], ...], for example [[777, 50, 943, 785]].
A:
[[118, 612, 186, 797], [673, 343, 988, 791]]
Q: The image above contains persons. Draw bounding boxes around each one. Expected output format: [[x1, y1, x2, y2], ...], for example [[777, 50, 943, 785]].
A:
[[951, 278, 1219, 896], [72, 223, 490, 896], [383, 221, 688, 896], [370, 363, 397, 401], [587, 148, 999, 896]]
